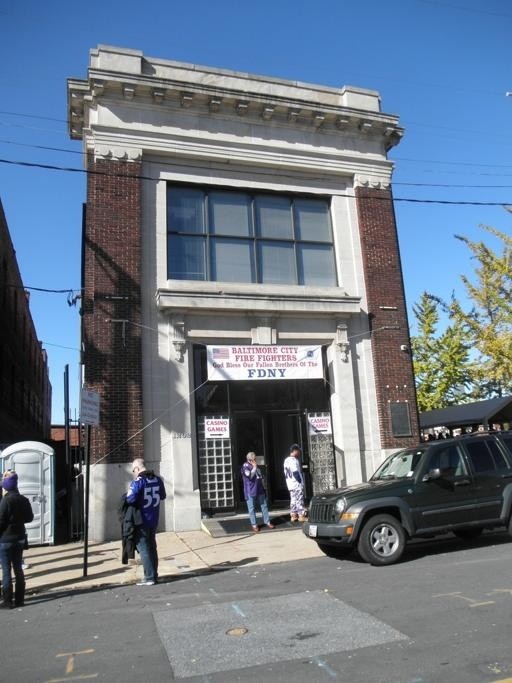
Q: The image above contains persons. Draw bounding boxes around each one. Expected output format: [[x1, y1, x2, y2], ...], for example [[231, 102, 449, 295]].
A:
[[239, 451, 275, 532], [125, 457, 167, 585], [0, 474, 35, 609], [0, 467, 33, 571], [418, 422, 512, 442], [282, 444, 307, 523]]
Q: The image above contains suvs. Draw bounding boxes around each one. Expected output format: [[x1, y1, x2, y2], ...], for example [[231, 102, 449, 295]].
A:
[[300, 427, 510, 567]]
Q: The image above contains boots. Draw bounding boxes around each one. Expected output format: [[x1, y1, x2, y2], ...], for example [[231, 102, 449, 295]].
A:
[[290, 512, 309, 523], [0, 583, 13, 609], [13, 581, 26, 606]]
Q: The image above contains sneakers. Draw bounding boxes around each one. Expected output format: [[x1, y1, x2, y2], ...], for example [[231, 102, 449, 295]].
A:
[[266, 524, 274, 529], [136, 580, 155, 586], [254, 527, 260, 532]]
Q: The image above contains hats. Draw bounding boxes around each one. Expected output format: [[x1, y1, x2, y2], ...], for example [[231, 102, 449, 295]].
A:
[[2, 474, 17, 491]]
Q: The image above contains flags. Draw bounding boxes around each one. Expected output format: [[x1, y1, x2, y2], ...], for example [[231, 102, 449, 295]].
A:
[[212, 347, 230, 359]]
